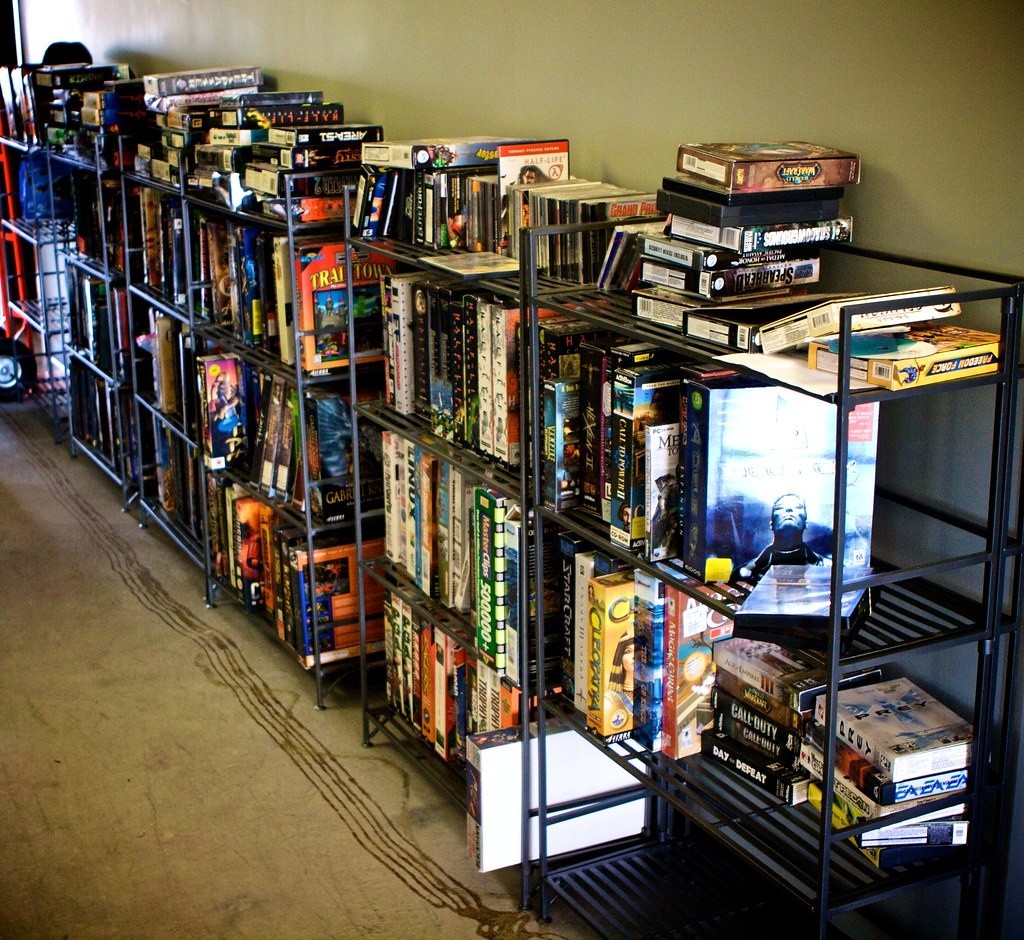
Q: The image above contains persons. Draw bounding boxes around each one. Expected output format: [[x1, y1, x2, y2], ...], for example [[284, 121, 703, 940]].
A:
[[501, 164, 553, 237]]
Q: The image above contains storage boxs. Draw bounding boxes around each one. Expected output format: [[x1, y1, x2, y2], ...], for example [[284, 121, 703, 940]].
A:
[[0, 62, 990, 875]]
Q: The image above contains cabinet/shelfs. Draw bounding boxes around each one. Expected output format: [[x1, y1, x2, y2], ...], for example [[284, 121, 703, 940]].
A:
[[178, 153, 387, 714], [341, 181, 662, 910], [524, 220, 1024, 940], [117, 133, 236, 602], [0, 116, 75, 443], [45, 120, 159, 514]]
